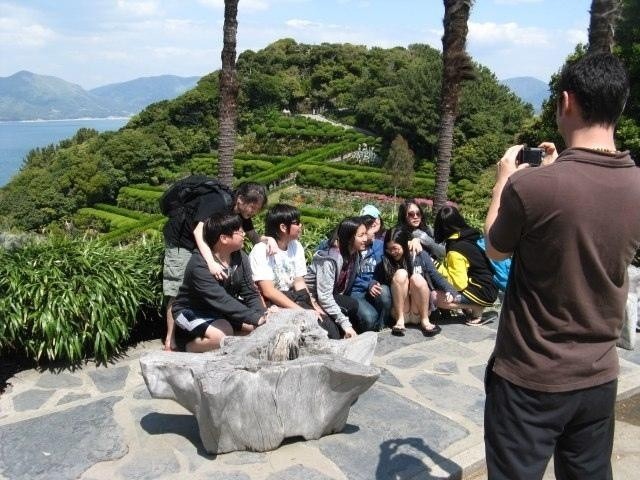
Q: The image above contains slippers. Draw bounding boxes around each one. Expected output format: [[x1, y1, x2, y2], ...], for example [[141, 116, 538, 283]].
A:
[[465, 310, 498, 326]]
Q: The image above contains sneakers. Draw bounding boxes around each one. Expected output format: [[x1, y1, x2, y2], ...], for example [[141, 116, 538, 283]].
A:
[[391, 325, 406, 332], [420, 321, 442, 336]]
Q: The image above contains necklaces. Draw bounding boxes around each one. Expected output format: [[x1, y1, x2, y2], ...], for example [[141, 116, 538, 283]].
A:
[[586, 146, 621, 156]]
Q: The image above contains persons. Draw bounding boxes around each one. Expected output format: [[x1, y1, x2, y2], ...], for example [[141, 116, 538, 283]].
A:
[[162, 182, 341, 353], [306, 171, 514, 356], [479, 50, 640, 480]]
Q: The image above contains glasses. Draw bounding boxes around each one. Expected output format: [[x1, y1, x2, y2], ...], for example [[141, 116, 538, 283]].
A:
[[386, 246, 404, 253], [292, 219, 301, 226], [232, 229, 245, 236], [406, 210, 421, 218]]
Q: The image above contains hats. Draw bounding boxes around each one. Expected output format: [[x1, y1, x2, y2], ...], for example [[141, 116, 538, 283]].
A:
[[359, 204, 382, 218]]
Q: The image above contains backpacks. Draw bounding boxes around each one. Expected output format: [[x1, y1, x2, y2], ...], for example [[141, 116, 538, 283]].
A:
[[157, 175, 234, 211]]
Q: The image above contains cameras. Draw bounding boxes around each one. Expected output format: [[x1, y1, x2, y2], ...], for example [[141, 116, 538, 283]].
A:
[[517, 149, 545, 166]]
